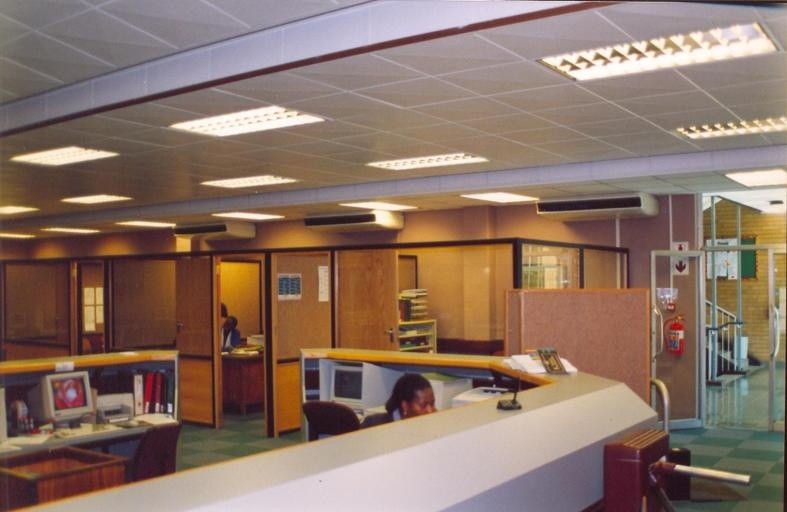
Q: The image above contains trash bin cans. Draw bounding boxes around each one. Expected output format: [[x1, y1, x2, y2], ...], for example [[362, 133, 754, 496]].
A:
[[666, 446, 690, 501]]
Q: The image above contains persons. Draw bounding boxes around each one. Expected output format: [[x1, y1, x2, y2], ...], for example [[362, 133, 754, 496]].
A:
[[222, 315, 243, 353], [358, 373, 437, 431]]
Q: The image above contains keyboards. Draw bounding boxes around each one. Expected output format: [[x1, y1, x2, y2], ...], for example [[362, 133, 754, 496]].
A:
[[54, 423, 119, 439]]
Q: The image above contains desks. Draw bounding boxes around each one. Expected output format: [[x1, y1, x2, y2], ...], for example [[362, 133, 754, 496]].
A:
[[0, 412, 177, 465]]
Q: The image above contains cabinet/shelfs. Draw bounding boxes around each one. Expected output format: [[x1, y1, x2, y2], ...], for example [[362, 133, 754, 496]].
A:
[[222, 348, 263, 415], [399, 319, 437, 356]]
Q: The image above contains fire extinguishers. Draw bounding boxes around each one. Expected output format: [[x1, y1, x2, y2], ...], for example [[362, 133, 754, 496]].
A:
[[663, 313, 686, 358]]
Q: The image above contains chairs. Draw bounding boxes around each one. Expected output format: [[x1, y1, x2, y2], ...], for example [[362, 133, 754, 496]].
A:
[[123, 424, 181, 482], [302, 400, 358, 442]]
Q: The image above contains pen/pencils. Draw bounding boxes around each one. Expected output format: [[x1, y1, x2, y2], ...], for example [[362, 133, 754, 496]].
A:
[[513, 376, 520, 402]]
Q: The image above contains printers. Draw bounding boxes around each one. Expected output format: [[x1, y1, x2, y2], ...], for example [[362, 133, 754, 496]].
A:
[[97, 392, 134, 424]]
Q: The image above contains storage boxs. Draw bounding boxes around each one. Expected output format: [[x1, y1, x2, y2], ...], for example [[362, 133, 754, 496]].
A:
[[1, 441, 128, 512]]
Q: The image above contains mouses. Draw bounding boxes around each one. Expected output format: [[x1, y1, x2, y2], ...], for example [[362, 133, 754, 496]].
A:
[[119, 420, 137, 427]]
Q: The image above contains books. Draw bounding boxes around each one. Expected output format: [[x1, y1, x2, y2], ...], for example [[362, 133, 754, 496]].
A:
[[130, 412, 177, 427], [116, 365, 175, 413], [399, 288, 427, 319], [506, 346, 577, 375]]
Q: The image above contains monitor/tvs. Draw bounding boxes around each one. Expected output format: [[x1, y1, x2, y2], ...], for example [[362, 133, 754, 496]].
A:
[[27, 371, 93, 429], [330, 366, 363, 408]]
